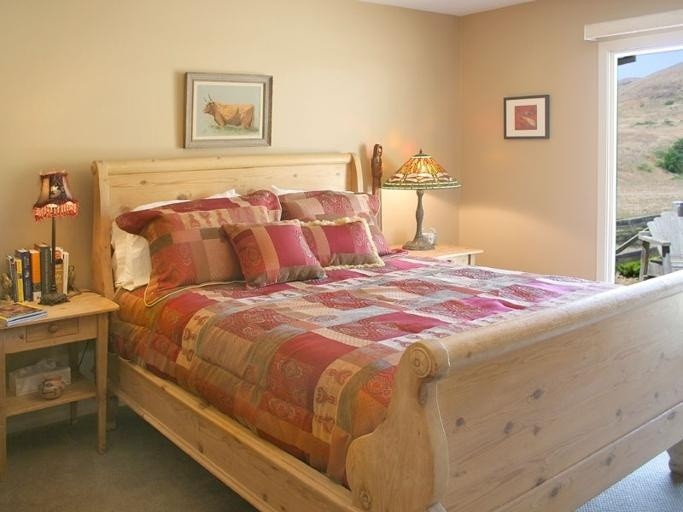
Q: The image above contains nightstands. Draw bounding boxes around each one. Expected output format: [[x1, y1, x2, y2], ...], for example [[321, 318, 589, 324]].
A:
[[389, 245, 484, 265], [1, 289, 120, 461]]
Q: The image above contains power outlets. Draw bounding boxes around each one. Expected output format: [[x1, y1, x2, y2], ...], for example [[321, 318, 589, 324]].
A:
[[422, 232, 435, 246]]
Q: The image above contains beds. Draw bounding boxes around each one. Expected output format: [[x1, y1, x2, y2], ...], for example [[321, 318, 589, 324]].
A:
[[91, 152, 683, 512]]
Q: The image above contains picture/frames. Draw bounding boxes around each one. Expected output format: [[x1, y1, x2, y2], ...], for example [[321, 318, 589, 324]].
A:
[[504, 94, 550, 139], [184, 72, 272, 148]]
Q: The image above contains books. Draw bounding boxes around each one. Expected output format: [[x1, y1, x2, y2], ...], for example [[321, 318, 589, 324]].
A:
[[0, 240, 73, 302], [6, 301, 48, 327]]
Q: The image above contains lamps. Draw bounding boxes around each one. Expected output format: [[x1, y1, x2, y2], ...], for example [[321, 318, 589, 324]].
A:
[[381, 149, 463, 251], [32, 171, 80, 306]]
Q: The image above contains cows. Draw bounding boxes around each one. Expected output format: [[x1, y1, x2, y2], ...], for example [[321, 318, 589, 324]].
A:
[[201, 93, 255, 131]]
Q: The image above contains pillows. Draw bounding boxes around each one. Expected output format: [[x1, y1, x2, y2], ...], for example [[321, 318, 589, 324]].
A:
[[107, 190, 283, 307], [279, 190, 396, 266], [301, 217, 385, 270], [221, 219, 328, 291], [111, 189, 242, 292]]
